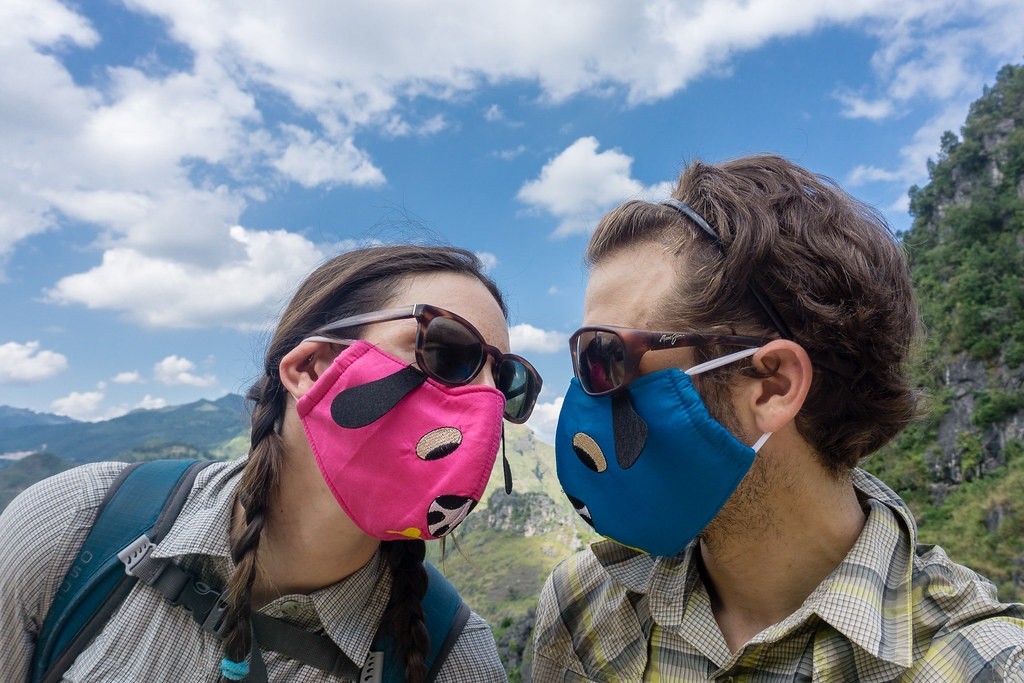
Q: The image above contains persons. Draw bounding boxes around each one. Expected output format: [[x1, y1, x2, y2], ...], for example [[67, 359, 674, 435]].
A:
[[0, 245, 542, 683], [529, 154, 1024, 683]]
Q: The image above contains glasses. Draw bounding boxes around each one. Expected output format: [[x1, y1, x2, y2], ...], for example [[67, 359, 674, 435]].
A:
[[306, 302, 543, 424], [567, 325, 776, 398]]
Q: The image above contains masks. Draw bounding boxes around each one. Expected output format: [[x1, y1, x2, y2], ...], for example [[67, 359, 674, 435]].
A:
[[293, 336, 508, 542], [557, 347, 777, 558]]
